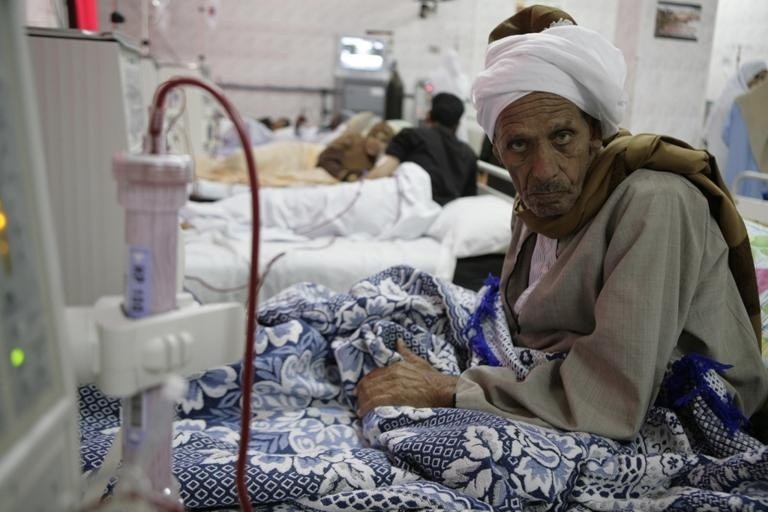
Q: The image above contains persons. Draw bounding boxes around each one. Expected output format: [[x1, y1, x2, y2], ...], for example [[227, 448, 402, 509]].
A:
[[315, 122, 395, 182], [317, 115, 343, 133], [352, 4, 768, 447], [261, 116, 290, 131], [384, 60, 403, 121], [362, 92, 478, 207]]
